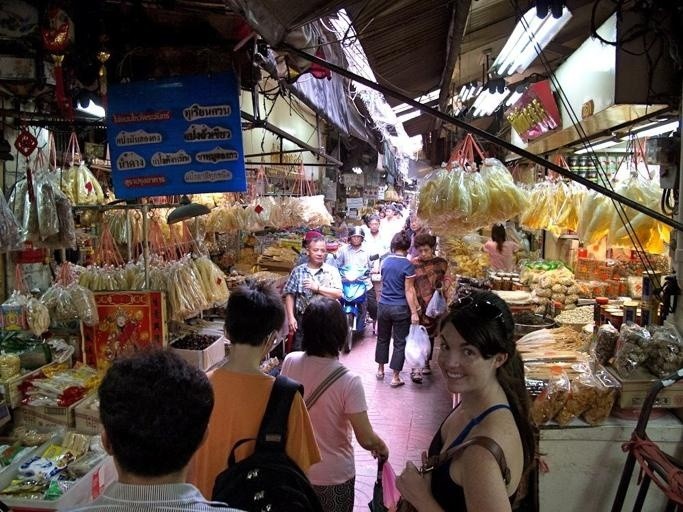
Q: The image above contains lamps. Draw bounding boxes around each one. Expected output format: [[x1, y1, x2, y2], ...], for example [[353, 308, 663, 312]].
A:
[[75, 195, 212, 227], [75, 94, 105, 123], [482, 2, 573, 81], [616, 115, 678, 139], [458, 79, 530, 120], [572, 136, 621, 155]]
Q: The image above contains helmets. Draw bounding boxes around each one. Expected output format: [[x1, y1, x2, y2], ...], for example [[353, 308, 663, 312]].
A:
[[347, 225, 364, 242]]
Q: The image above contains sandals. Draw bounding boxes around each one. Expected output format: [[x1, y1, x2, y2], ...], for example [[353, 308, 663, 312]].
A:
[[376, 369, 384, 378], [410, 371, 422, 382], [422, 362, 432, 374], [390, 377, 404, 388]]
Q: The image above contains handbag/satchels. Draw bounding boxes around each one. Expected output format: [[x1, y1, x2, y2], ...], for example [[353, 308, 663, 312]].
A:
[[396, 436, 511, 512]]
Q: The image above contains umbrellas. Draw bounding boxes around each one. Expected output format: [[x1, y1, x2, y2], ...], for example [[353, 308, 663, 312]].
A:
[[367, 454, 386, 512]]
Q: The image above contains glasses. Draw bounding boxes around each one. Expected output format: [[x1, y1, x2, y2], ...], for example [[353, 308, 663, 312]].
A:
[[272, 330, 284, 345], [450, 296, 506, 329]]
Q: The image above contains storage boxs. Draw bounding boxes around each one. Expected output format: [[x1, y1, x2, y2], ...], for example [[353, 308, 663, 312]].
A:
[[0, 352, 119, 512], [605, 359, 682, 410], [169, 331, 226, 377], [222, 235, 302, 307]]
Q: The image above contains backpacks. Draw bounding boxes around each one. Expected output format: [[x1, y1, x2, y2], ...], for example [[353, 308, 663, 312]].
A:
[[211, 375, 323, 512]]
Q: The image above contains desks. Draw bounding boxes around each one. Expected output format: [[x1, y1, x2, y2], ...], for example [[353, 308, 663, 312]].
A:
[[533, 415, 682, 512]]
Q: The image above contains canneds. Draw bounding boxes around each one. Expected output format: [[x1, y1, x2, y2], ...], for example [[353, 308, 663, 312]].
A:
[[489, 272, 520, 291], [593, 270, 664, 332]]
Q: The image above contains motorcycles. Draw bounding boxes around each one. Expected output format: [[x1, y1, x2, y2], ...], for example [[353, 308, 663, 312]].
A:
[[336, 253, 380, 354]]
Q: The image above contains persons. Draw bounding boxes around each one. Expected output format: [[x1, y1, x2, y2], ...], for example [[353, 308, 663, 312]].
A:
[[279, 198, 450, 388], [185, 280, 322, 500], [393, 290, 539, 511], [279, 297, 387, 511], [56, 348, 253, 511]]
